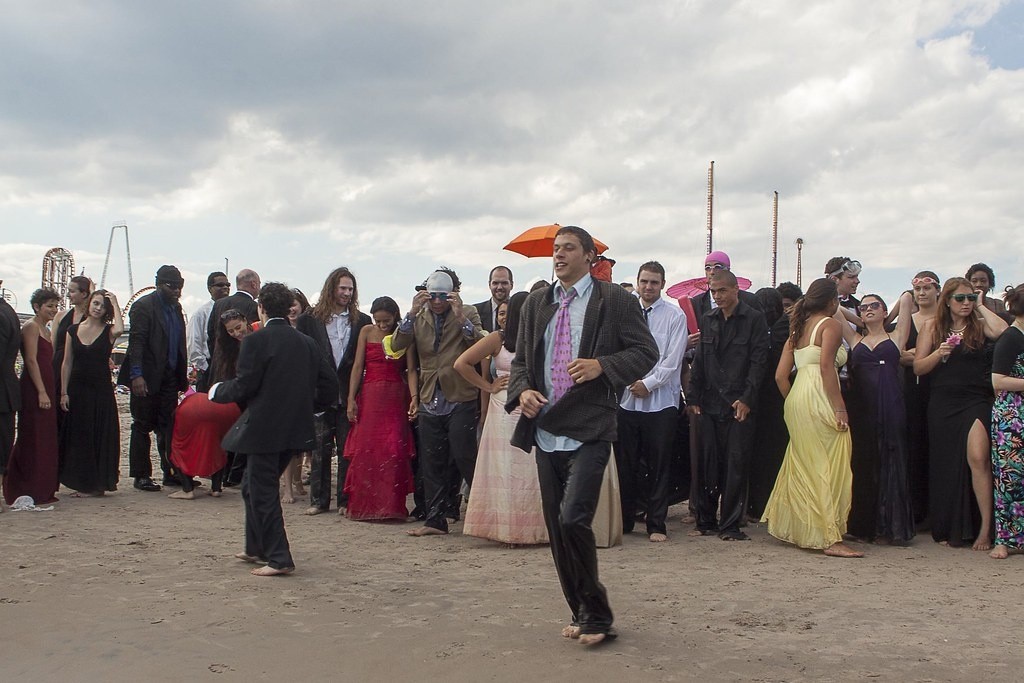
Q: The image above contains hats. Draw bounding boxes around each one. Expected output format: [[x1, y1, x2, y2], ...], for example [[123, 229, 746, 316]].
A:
[[704, 252, 730, 267], [157, 266, 184, 283], [415, 281, 428, 292], [428, 272, 453, 294]]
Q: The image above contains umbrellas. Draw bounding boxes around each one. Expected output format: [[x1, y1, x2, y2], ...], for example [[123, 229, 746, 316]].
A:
[[666, 276, 753, 299], [501, 221, 609, 283]]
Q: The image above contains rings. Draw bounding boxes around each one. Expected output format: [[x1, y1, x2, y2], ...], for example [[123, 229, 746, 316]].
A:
[[46, 404, 48, 407]]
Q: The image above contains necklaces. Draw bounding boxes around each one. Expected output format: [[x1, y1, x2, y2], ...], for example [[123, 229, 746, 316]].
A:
[[949, 325, 966, 336], [36, 317, 50, 337]]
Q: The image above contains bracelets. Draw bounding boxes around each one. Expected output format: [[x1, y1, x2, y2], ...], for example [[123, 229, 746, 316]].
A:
[[61, 394, 68, 396], [411, 394, 417, 398], [976, 304, 983, 309], [836, 410, 846, 412]]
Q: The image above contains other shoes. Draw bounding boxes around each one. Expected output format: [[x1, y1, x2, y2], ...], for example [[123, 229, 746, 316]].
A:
[[163, 478, 201, 488], [134, 476, 161, 491]]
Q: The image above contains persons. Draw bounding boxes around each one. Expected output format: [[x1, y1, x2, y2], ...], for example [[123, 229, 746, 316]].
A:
[[505, 225, 660, 646], [618, 251, 1024, 559], [116, 265, 550, 544], [208, 282, 340, 576], [0, 276, 125, 515]]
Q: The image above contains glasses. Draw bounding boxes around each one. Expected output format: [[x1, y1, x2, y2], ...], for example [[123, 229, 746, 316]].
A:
[[290, 288, 300, 295], [211, 282, 231, 287], [859, 302, 881, 311], [428, 292, 450, 301], [949, 293, 978, 302], [912, 277, 938, 285], [220, 312, 240, 319], [841, 260, 862, 278], [704, 265, 725, 273], [164, 281, 183, 290]]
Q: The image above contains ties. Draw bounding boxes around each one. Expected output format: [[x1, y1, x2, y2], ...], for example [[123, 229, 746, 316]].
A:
[[495, 306, 501, 330], [551, 289, 578, 406], [642, 307, 652, 325]]
